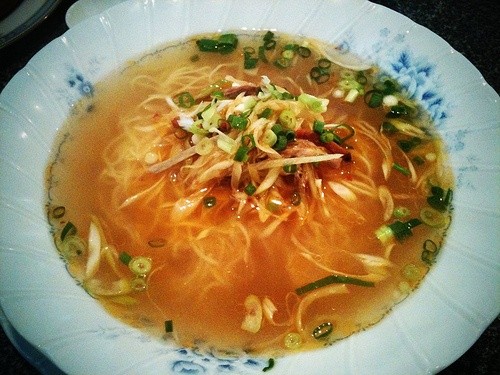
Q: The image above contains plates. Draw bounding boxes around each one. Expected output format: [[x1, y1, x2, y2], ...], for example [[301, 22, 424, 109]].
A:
[[0, 0, 500, 375]]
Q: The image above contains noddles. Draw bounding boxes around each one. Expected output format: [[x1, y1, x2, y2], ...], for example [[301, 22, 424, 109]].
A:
[[44, 30, 453, 352]]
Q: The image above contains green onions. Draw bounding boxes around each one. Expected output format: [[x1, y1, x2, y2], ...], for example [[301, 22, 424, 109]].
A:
[[49, 30, 451, 352]]
